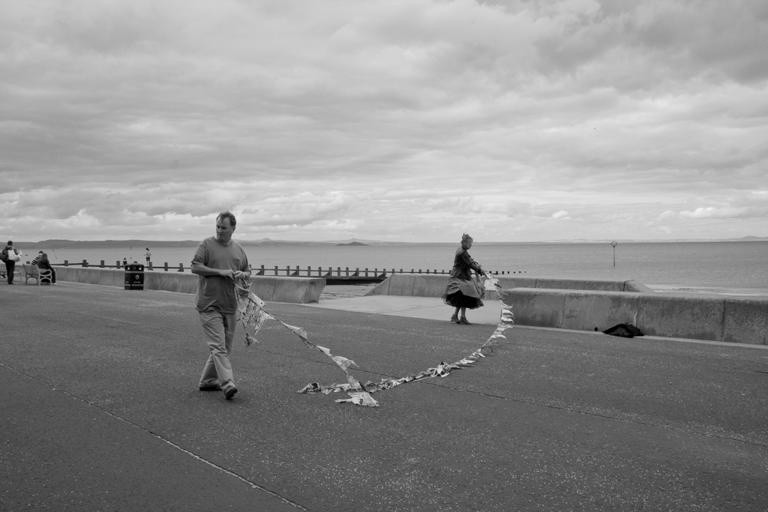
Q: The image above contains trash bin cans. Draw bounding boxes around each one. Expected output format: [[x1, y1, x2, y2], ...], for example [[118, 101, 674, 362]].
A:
[[124, 263, 145, 290]]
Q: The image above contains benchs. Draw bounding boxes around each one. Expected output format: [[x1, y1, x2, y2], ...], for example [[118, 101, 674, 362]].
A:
[[0, 263, 11, 278], [22, 260, 54, 287]]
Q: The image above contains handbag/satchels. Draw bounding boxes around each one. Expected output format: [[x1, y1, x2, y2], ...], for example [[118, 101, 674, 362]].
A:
[[7, 249, 20, 261]]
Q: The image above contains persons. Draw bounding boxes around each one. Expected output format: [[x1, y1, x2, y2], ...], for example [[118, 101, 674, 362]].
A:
[[36, 252, 57, 285], [0, 239, 18, 286], [439, 233, 493, 329], [30, 249, 44, 266], [189, 211, 252, 400], [142, 246, 154, 268]]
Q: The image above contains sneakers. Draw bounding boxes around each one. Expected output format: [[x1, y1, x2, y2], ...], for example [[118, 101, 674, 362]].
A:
[[223, 382, 238, 400]]
